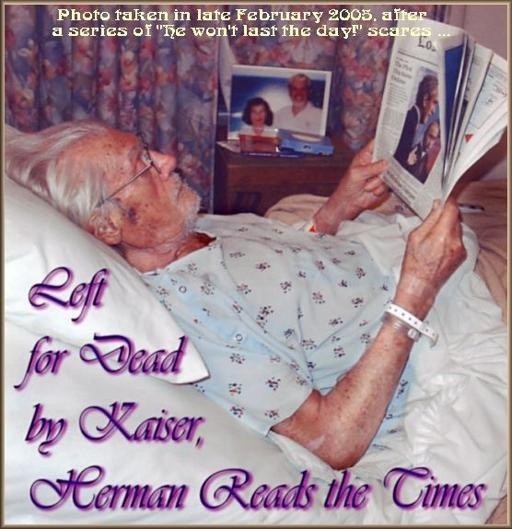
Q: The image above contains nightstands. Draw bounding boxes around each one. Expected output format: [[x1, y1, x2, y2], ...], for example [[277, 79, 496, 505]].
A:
[[212, 131, 357, 220]]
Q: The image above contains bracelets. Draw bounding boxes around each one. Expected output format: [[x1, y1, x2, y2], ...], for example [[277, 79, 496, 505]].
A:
[[379, 300, 439, 348]]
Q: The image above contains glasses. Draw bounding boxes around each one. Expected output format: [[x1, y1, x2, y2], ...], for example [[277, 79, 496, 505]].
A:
[[94, 144, 164, 210]]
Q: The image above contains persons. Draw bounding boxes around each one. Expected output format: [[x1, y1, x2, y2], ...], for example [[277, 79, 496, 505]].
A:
[[392, 76, 444, 180], [2, 103, 471, 471], [240, 72, 324, 141]]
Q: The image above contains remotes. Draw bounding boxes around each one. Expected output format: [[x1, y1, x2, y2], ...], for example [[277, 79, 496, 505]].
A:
[[456, 201, 487, 215]]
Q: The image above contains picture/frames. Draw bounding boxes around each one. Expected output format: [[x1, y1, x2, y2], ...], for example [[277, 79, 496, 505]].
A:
[[230, 65, 333, 143]]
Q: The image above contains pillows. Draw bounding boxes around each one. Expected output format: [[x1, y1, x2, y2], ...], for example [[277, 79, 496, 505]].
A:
[[1, 121, 211, 386]]
